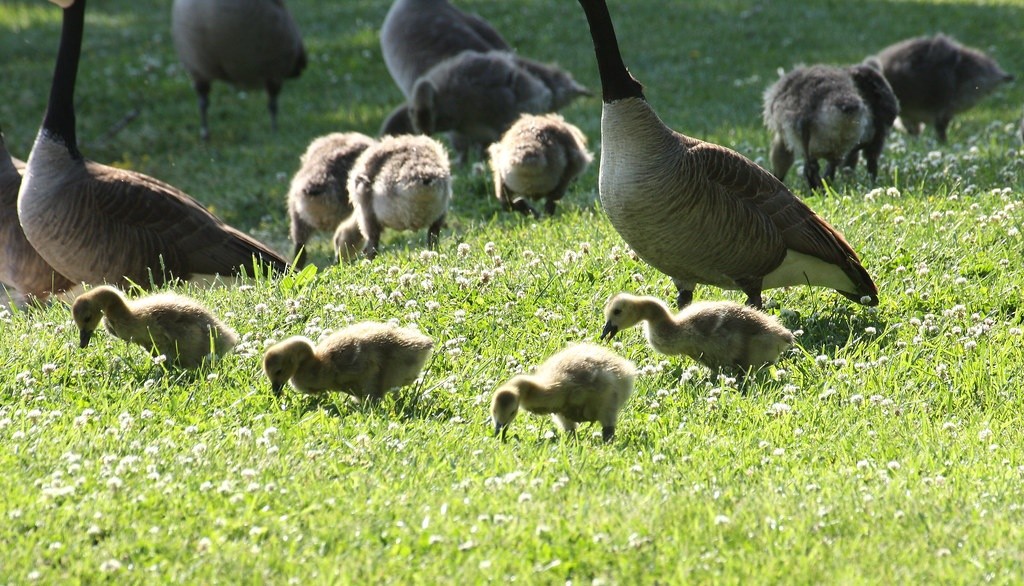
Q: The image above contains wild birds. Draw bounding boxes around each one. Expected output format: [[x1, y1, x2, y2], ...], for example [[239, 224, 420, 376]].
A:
[[577, 0, 879, 323], [599, 291, 793, 398], [333, 131, 458, 267], [264, 322, 434, 411], [378, 0, 593, 148], [16, 0, 305, 293], [490, 344, 638, 445], [1, 130, 75, 311], [486, 112, 598, 218], [73, 283, 237, 372], [293, 130, 378, 272], [764, 33, 1015, 188], [168, 1, 308, 142]]
